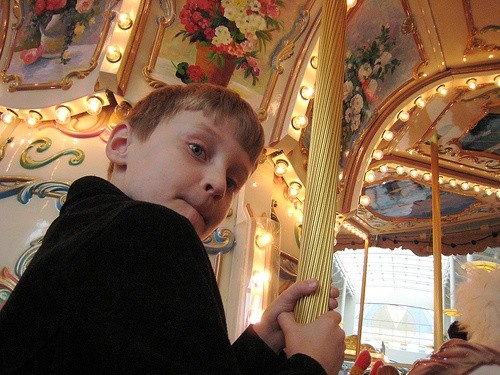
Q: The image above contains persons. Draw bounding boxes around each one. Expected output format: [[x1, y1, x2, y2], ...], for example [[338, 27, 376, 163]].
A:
[[0, 84, 346, 375], [405, 263, 500, 375]]
